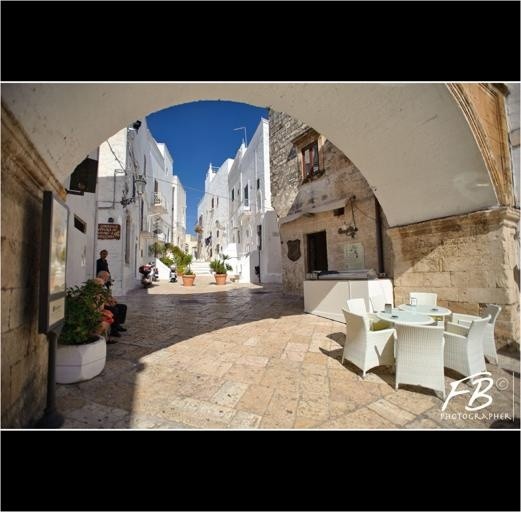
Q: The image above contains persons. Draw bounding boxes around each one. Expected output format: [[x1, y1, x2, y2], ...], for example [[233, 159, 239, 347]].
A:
[[96, 249, 111, 288], [98, 271, 127, 337], [90, 278, 118, 344]]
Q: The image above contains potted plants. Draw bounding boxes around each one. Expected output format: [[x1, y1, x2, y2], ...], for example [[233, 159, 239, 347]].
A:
[[54, 278, 118, 385], [214, 260, 227, 285], [182, 269, 195, 287]]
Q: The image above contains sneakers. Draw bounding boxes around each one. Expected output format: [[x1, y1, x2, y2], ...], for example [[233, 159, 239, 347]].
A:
[[117, 326, 126, 332], [111, 331, 121, 337], [107, 340, 117, 344]]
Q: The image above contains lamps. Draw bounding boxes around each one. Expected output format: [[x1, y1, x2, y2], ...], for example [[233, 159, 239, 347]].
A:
[[120, 174, 146, 208], [337, 222, 358, 239]]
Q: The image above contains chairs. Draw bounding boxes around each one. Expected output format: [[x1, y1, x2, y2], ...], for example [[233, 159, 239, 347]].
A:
[[341, 291, 502, 401]]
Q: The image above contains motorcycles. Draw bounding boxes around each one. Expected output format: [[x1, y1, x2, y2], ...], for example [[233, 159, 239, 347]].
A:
[[150, 262, 159, 281], [138, 264, 156, 290], [169, 265, 177, 282]]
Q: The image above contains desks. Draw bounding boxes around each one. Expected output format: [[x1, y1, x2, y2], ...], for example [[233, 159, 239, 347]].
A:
[[303, 277, 394, 324]]
